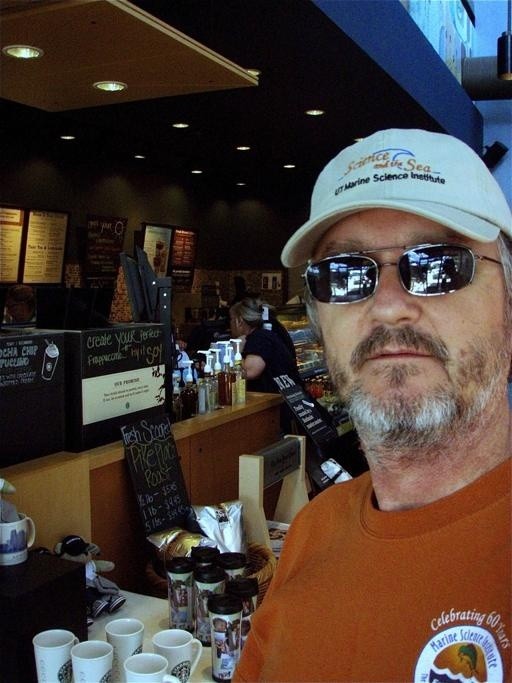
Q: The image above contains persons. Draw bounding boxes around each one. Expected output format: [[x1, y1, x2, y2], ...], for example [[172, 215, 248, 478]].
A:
[[231, 129, 512, 683], [230, 300, 296, 432], [6, 285, 36, 324]]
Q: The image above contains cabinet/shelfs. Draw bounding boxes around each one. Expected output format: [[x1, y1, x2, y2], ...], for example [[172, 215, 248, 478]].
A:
[[277, 305, 360, 451]]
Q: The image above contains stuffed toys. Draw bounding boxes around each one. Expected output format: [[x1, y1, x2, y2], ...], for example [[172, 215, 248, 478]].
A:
[[55, 535, 126, 619]]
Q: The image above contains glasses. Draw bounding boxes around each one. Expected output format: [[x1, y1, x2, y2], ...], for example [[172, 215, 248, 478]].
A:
[[301, 244, 503, 304]]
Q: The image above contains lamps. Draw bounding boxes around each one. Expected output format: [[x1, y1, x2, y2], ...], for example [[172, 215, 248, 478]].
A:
[[1, 44, 45, 60], [92, 80, 128, 94]]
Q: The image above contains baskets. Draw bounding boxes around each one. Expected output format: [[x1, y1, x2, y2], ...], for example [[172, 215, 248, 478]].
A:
[[146, 539, 275, 615]]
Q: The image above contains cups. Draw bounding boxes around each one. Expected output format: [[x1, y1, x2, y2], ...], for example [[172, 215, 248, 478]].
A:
[[207, 593, 245, 678], [41, 351, 58, 380], [195, 565, 226, 647], [153, 629, 203, 683], [191, 546, 220, 567], [218, 552, 246, 584], [123, 652, 183, 683], [70, 641, 114, 683], [32, 629, 79, 683], [166, 557, 195, 632], [0, 513, 37, 567], [104, 618, 145, 682]]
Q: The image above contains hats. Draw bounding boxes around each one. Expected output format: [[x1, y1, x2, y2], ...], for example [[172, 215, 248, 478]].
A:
[[280, 128, 512, 267]]
